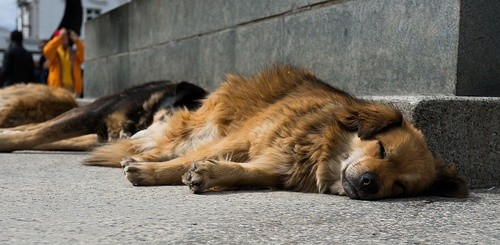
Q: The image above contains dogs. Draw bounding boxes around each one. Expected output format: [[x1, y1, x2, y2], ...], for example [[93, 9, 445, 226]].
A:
[[82, 64, 470, 201], [0, 80, 211, 153], [0, 82, 77, 130]]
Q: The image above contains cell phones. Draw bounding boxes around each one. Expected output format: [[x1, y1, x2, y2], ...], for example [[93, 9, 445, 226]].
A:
[[65, 30, 71, 35]]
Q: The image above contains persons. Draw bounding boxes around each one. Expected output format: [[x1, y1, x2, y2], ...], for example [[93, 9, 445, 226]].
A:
[[0, 30, 37, 89], [42, 27, 85, 97]]
